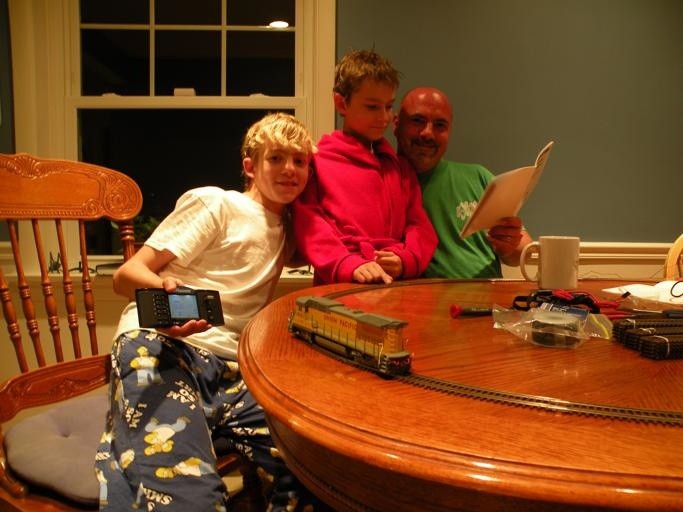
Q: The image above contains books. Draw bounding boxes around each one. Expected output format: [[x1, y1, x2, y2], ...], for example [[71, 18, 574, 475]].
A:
[[458, 140, 555, 241]]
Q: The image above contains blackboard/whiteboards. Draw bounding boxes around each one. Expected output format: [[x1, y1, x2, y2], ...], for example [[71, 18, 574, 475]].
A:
[[311, 0, 683, 266]]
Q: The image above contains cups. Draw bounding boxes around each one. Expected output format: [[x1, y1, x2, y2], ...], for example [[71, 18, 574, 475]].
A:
[[521, 236, 580, 291]]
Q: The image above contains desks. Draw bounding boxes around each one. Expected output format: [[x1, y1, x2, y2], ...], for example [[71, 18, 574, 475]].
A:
[[237, 277, 683, 510]]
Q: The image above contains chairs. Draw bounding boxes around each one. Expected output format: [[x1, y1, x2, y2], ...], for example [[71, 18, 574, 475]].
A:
[[663, 233, 683, 280], [0, 152, 269, 511]]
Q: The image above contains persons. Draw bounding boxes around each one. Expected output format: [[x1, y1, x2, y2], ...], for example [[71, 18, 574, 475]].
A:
[[390, 85, 536, 280], [91, 113, 334, 510], [288, 43, 441, 287]]
